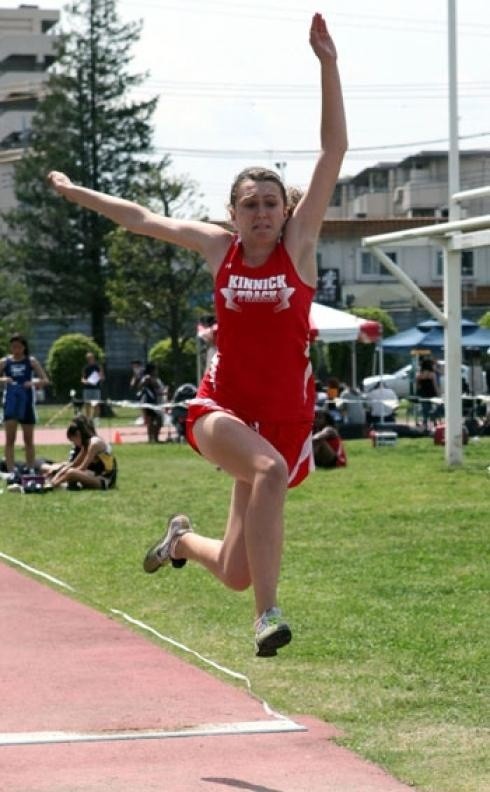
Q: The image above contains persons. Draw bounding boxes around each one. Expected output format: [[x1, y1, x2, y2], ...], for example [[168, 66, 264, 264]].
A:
[[80, 351, 105, 416], [170, 383, 197, 442], [52, 418, 118, 489], [311, 410, 347, 467], [0, 332, 51, 471], [130, 360, 169, 443], [46, 11, 349, 651], [40, 418, 96, 488], [313, 376, 400, 439], [415, 359, 441, 434]]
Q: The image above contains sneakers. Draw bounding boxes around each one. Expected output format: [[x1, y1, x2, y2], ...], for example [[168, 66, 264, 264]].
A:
[[142, 513, 192, 574], [254, 606, 293, 658]]
